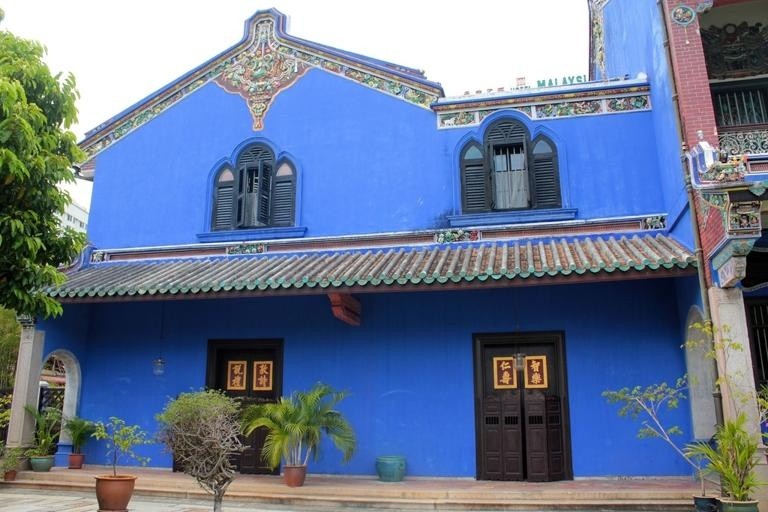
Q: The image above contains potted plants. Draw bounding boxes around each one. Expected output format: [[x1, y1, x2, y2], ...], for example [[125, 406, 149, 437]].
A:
[[81, 414, 160, 512], [1, 439, 26, 481], [608, 325, 767, 512], [21, 400, 68, 472], [62, 416, 96, 469], [678, 411, 766, 512], [239, 380, 355, 487]]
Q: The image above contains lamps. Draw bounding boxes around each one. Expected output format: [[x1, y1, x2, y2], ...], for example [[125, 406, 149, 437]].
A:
[[152, 300, 166, 376]]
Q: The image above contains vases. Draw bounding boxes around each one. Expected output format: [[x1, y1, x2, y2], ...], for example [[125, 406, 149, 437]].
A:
[[372, 455, 406, 483]]
